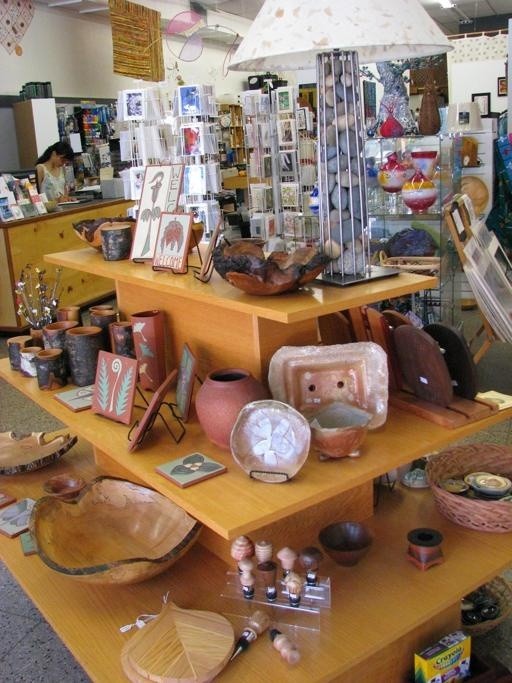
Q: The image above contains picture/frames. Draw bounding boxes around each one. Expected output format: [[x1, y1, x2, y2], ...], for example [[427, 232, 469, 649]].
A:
[[52, 385, 94, 413], [174, 342, 204, 423], [154, 451, 226, 489], [129, 162, 185, 264], [151, 212, 202, 275], [91, 350, 149, 426], [0, 492, 38, 556], [192, 215, 223, 282], [126, 370, 186, 454], [498, 77, 507, 97], [471, 93, 490, 118]]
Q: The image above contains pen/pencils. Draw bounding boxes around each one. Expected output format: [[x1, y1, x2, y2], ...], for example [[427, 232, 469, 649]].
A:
[[59, 192, 65, 198]]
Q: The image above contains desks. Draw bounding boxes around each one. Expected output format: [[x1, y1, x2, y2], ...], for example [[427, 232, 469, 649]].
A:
[[1, 199, 137, 334]]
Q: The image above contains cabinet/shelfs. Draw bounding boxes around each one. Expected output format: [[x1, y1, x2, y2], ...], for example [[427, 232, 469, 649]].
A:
[[215, 103, 247, 167], [0, 237, 512, 682]]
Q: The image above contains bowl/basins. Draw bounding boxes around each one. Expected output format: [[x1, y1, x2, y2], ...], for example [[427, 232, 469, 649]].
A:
[[225, 239, 268, 251], [42, 474, 86, 498], [308, 408, 372, 458], [28, 475, 202, 586], [73, 213, 205, 252], [319, 519, 375, 566]]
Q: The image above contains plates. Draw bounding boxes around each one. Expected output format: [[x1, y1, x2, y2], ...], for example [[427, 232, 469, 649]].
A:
[[230, 400, 312, 482]]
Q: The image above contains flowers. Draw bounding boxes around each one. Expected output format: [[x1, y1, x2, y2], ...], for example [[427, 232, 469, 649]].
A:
[[14, 265, 65, 328]]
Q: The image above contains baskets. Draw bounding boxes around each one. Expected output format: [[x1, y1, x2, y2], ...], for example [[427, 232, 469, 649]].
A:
[[421, 436, 511, 537]]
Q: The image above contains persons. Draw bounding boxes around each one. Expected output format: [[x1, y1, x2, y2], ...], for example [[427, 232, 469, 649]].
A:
[[34, 140, 78, 202]]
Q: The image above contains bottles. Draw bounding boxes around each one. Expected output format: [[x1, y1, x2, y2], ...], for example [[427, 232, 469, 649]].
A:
[[419, 81, 442, 136], [20, 82, 52, 100], [228, 534, 325, 664], [195, 367, 273, 449]]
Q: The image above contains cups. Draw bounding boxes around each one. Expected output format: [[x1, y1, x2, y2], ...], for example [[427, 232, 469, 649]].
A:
[[7, 305, 167, 392], [101, 225, 131, 261]]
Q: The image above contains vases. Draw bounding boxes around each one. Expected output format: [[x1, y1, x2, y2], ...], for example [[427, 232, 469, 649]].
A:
[[5, 304, 166, 393], [376, 150, 437, 214]]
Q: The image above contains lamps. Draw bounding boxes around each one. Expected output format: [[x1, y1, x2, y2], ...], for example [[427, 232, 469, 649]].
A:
[[440, 0, 455, 11], [227, 1, 457, 289]]
